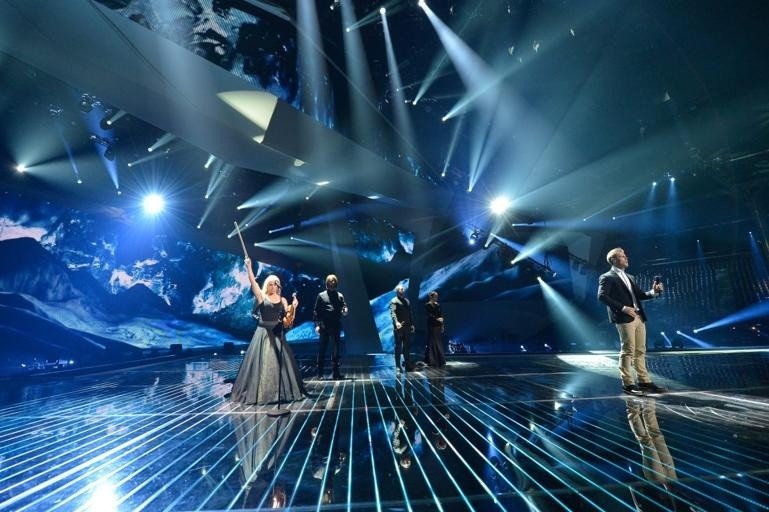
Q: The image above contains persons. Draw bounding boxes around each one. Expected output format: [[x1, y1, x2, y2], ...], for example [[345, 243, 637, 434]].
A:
[[312, 272, 349, 381], [594, 244, 667, 397], [424, 375, 451, 453], [308, 403, 348, 508], [231, 410, 293, 510], [415, 288, 448, 369], [227, 257, 307, 408], [389, 282, 417, 373], [389, 373, 417, 473], [621, 399, 701, 510]]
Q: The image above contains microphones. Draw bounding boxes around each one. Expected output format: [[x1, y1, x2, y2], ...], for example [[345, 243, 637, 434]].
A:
[[394, 321, 406, 332], [292, 292, 296, 318], [655, 276, 661, 298]]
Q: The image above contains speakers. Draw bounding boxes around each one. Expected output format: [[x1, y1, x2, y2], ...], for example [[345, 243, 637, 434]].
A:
[[223, 342, 233, 349], [170, 344, 182, 354]]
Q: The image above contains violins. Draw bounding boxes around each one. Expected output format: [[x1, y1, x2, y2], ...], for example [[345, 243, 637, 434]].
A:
[[282, 291, 296, 328]]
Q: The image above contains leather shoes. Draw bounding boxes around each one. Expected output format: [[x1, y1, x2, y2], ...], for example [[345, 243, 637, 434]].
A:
[[624, 385, 646, 396], [638, 383, 665, 393]]
